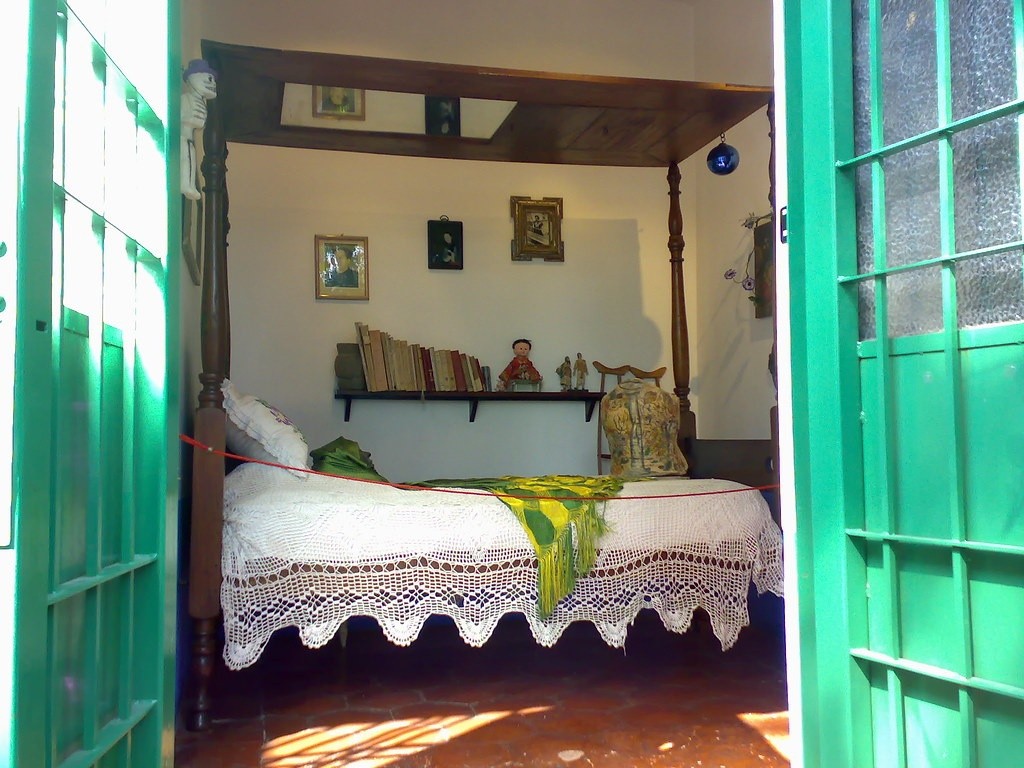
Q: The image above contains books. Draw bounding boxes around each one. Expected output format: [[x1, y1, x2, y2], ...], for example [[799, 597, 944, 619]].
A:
[[354, 322, 491, 392]]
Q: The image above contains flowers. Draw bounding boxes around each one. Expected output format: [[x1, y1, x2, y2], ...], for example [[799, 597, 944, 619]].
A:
[[724, 209, 775, 305]]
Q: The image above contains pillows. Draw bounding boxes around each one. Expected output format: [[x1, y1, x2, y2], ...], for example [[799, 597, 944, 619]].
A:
[[220, 378, 310, 481]]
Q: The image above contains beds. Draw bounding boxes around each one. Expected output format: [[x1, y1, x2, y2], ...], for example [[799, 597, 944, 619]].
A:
[[183, 38, 789, 730]]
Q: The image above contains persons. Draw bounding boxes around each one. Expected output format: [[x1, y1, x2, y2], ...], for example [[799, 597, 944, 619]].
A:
[[573, 353, 588, 390], [558, 356, 572, 390], [532, 215, 544, 247], [180, 59, 216, 198], [325, 247, 359, 287], [499, 339, 543, 388]]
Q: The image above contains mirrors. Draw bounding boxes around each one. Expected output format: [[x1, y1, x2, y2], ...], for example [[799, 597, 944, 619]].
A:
[[280, 81, 518, 139]]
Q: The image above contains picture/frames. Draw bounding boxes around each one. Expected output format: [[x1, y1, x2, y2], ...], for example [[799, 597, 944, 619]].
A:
[[312, 85, 365, 122], [752, 222, 773, 319], [314, 234, 370, 301], [424, 94, 460, 138], [510, 195, 565, 262], [427, 220, 463, 271]]
